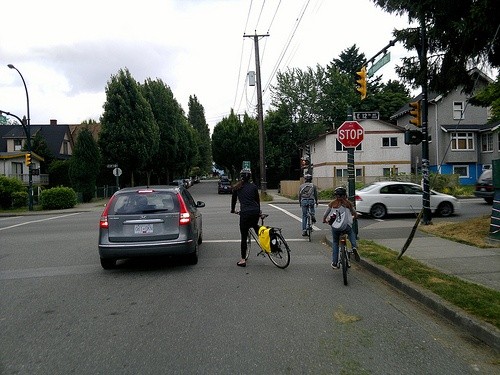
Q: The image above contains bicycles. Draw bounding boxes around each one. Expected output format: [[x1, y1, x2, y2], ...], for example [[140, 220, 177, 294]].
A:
[[234, 208, 292, 270], [303, 203, 317, 243], [322, 217, 356, 286]]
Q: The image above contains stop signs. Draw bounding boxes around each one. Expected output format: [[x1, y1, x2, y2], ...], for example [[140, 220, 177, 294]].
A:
[[336, 121, 364, 149]]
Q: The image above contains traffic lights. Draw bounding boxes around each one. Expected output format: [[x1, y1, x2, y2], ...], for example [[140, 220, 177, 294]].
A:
[[355, 66, 366, 100], [409, 102, 421, 127], [25, 154, 32, 167]]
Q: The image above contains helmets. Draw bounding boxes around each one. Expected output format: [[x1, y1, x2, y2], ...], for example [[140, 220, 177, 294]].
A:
[[334, 187, 347, 195], [240, 168, 251, 174], [305, 174, 312, 178]]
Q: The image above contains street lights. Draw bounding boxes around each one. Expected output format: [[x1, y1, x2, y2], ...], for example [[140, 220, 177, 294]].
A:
[[6, 64, 34, 211]]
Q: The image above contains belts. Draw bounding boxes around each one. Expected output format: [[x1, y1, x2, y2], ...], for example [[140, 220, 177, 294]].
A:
[[301, 198, 314, 202]]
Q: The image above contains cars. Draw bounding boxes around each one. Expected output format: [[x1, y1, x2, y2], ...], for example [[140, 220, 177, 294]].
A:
[[171, 175, 207, 189], [472, 169, 494, 205], [217, 175, 232, 194], [98, 184, 206, 268], [355, 181, 461, 220]]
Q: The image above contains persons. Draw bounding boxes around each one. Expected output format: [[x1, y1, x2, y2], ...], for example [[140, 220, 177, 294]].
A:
[[322, 187, 360, 269], [299, 174, 318, 236], [231, 168, 261, 267]]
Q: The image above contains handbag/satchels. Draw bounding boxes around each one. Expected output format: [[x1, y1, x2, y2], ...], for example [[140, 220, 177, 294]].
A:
[[258, 227, 279, 254], [330, 211, 348, 231], [300, 185, 314, 198]]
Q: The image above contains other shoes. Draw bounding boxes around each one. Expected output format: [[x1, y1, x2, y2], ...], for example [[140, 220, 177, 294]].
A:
[[302, 230, 307, 236], [311, 216, 317, 223], [352, 247, 360, 262], [331, 262, 337, 268], [237, 261, 246, 267]]
